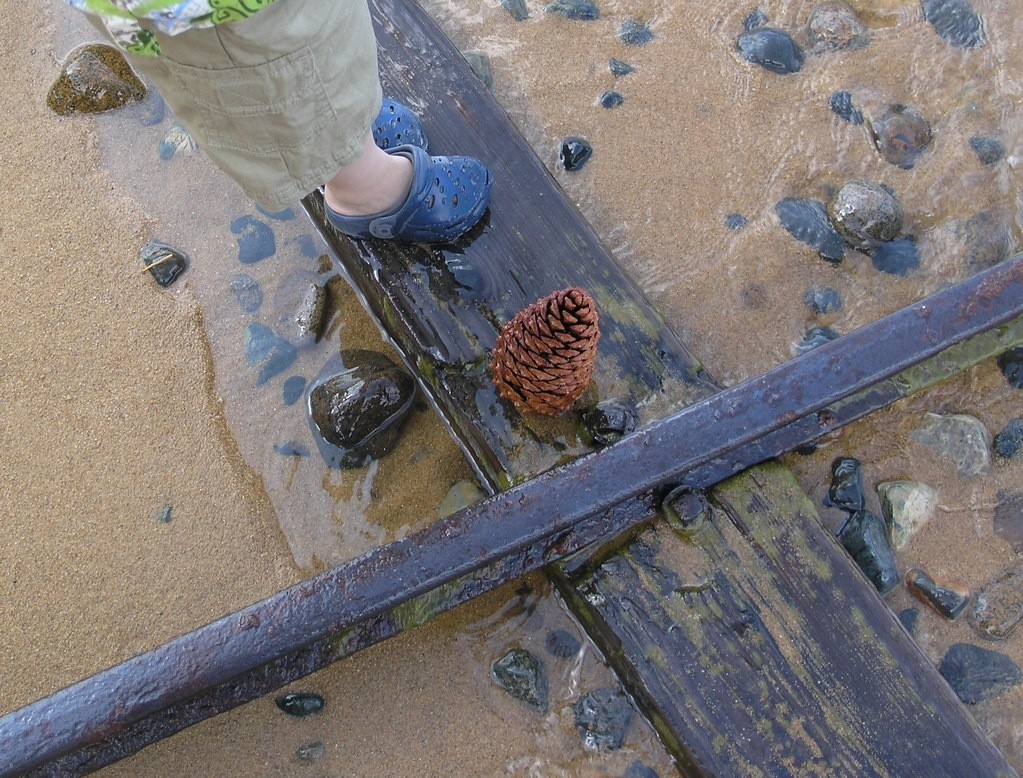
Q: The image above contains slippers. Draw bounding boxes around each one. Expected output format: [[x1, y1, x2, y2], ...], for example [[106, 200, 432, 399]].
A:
[[370, 96, 429, 153], [324, 142, 493, 243]]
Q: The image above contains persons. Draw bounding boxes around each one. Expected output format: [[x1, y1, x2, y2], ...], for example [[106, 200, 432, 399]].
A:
[[70, 0, 496, 245]]
